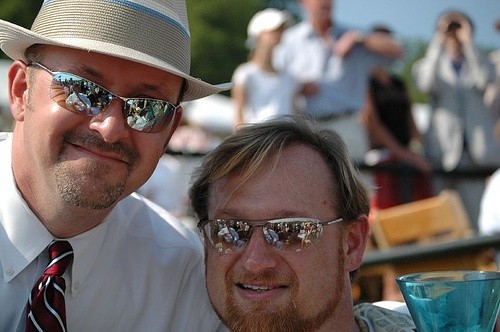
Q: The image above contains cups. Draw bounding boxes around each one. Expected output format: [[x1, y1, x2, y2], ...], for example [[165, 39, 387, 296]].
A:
[[395, 269, 500, 332]]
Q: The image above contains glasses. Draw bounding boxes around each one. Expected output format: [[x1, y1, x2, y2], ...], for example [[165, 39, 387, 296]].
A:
[[197, 216, 350, 256], [26, 61, 181, 134]]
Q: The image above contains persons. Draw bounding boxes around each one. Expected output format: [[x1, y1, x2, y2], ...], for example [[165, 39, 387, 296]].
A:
[[66, 81, 113, 115], [128, 100, 167, 131], [411, 10, 500, 241], [0, 0, 230, 332], [371, 25, 432, 210], [231, 7, 304, 131], [263, 220, 321, 252], [188, 115, 417, 332], [215, 218, 251, 247], [283, 1, 403, 127]]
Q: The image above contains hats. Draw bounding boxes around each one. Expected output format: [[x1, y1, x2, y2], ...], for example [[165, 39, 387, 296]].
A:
[[0, 0, 234, 102], [246, 7, 291, 39]]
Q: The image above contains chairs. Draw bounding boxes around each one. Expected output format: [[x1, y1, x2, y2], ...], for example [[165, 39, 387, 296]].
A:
[[370, 189, 498, 301]]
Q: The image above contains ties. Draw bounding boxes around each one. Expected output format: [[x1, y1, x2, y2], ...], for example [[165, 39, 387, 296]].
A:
[[24, 240, 74, 332]]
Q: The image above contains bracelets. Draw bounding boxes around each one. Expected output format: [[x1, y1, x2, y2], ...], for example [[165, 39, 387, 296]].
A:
[[357, 29, 366, 44]]
